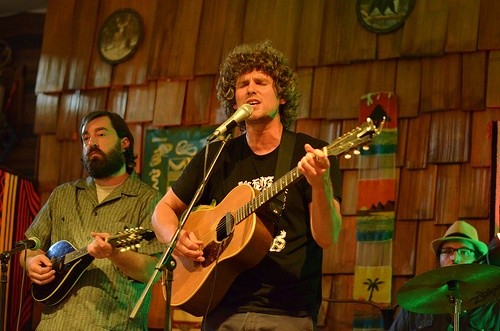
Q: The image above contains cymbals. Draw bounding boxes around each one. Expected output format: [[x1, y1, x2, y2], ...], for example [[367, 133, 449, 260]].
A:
[[396, 264, 500, 314]]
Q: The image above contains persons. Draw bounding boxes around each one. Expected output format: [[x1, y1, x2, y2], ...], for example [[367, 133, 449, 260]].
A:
[[151, 38, 344, 331], [393, 219, 500, 331], [19, 110, 161, 331]]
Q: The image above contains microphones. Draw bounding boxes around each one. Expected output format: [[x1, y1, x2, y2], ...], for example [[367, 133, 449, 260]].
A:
[[16, 237, 40, 250], [207, 103, 254, 142]]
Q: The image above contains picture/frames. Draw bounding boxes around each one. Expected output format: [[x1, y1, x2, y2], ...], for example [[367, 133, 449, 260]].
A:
[[357, 0, 414, 34], [97, 7, 144, 65]]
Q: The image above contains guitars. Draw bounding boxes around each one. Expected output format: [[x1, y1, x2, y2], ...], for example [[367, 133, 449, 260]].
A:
[[32, 227, 156, 306], [170, 118, 377, 316]]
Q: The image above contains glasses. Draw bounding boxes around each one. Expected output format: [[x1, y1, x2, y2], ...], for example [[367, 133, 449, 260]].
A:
[[440, 245, 477, 260]]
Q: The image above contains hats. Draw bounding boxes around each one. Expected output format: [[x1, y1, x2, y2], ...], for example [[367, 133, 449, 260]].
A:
[[430, 219, 489, 259]]
